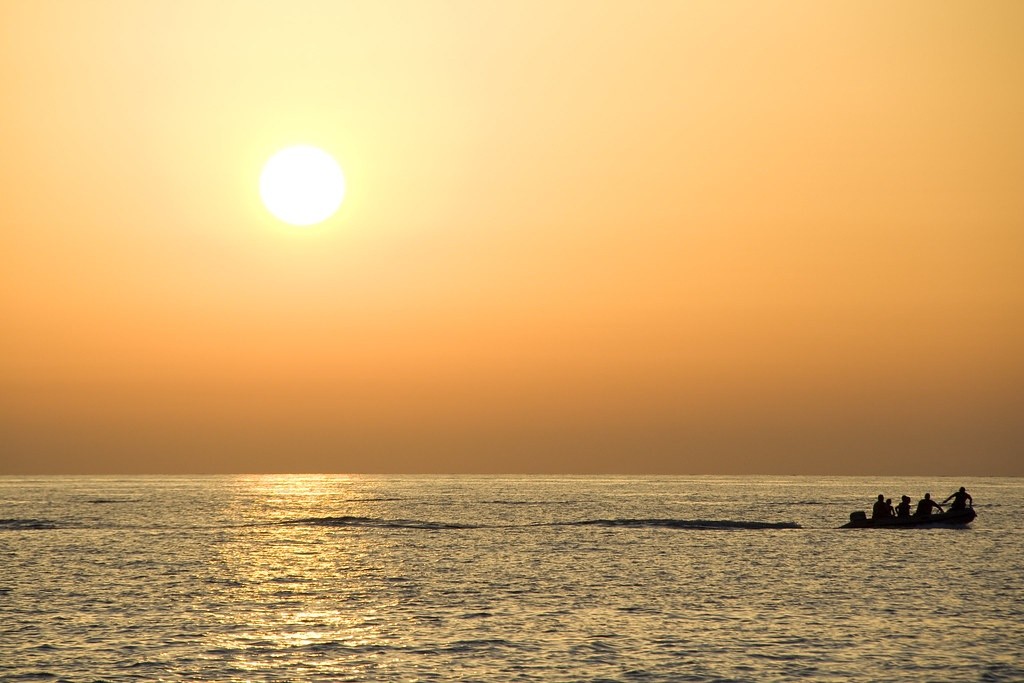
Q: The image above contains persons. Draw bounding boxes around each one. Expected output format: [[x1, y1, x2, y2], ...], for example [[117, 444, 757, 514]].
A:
[[872, 487, 972, 520]]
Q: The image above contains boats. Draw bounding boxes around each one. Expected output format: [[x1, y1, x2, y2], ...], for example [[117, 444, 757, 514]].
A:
[[838, 508, 978, 526]]
